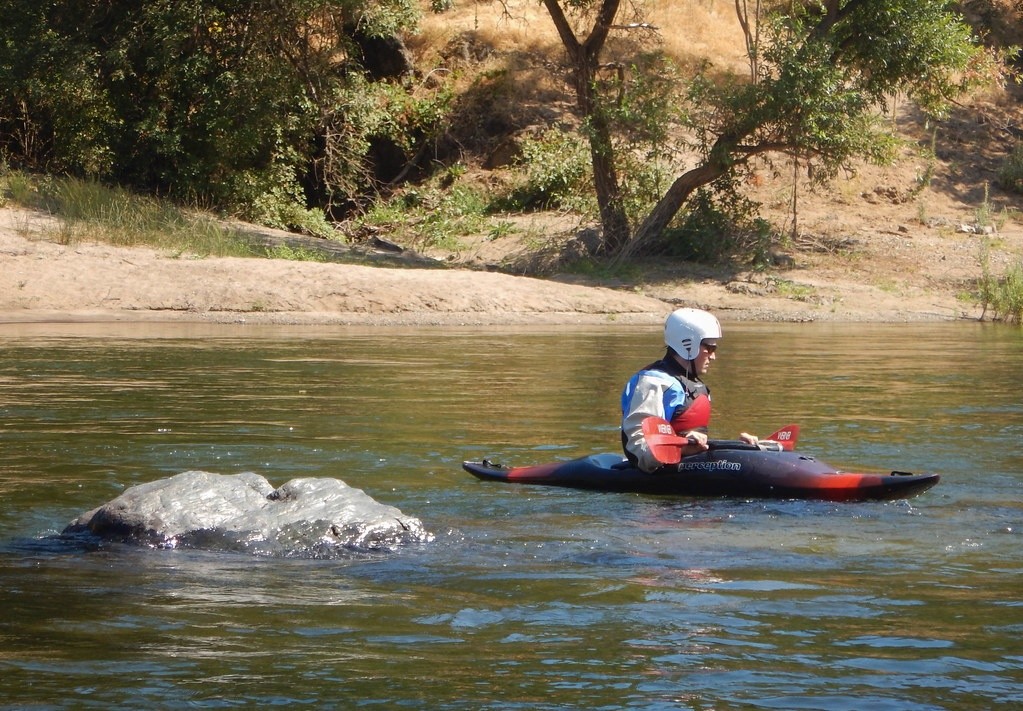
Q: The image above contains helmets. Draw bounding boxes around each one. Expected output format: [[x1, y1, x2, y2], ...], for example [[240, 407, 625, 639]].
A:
[[665, 308, 722, 360]]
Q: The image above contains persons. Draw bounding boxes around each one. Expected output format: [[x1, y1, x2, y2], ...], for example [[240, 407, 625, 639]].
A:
[[620, 308, 759, 475]]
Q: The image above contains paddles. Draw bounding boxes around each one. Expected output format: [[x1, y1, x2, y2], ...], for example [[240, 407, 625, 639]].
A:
[[640, 416, 801, 464]]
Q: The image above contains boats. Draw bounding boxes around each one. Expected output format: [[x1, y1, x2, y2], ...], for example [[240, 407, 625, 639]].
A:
[[463, 446, 942, 503]]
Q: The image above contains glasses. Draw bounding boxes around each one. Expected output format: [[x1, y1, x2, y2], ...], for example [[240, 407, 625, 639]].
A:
[[700, 343, 717, 354]]
